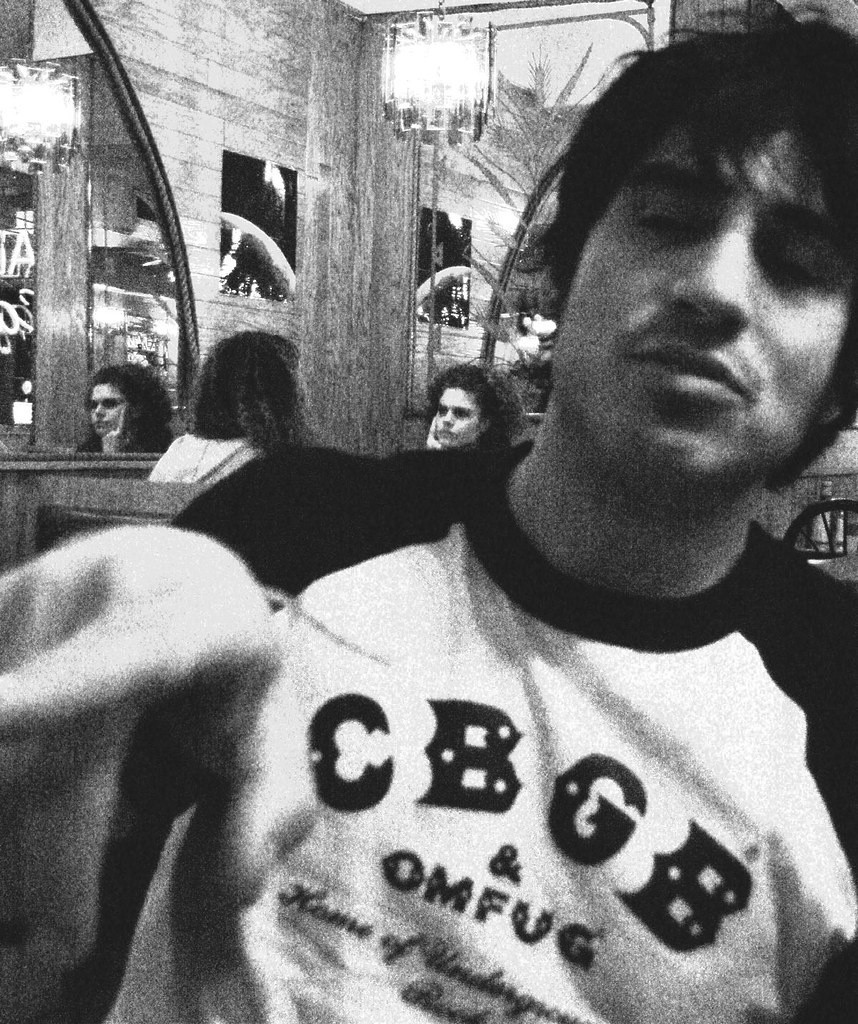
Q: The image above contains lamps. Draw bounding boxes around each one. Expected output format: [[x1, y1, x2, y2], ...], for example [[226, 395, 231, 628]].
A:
[[380, 13, 497, 408], [0, 57, 92, 179]]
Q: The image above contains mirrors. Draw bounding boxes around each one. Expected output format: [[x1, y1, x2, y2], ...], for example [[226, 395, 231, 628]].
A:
[[405, 0, 674, 421], [0, 0, 200, 465]]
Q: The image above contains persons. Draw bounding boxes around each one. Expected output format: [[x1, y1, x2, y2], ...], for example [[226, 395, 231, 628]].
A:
[[425, 361, 528, 452], [0, 6, 858, 1024], [75, 364, 174, 452], [142, 327, 305, 487]]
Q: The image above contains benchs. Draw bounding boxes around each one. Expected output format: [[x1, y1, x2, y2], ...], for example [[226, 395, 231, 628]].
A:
[[35, 475, 215, 554]]
